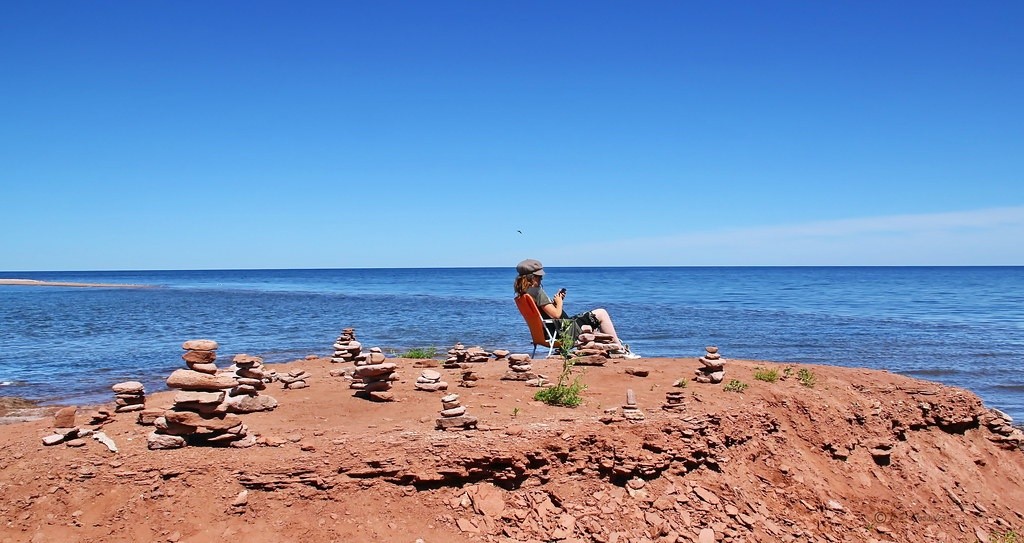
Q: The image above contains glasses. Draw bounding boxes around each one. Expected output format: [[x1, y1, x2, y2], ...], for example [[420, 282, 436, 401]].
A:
[[534, 275, 542, 277]]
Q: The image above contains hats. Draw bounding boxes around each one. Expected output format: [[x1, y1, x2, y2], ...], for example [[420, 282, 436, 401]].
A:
[[517, 258, 545, 275]]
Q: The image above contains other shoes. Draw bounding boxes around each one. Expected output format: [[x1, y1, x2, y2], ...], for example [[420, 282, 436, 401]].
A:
[[609, 344, 641, 359]]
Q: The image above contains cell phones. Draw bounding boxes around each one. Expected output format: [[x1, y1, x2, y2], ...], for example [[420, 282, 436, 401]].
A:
[[559, 288, 566, 296]]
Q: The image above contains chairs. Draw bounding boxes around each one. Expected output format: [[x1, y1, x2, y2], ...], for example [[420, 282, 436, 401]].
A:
[[514, 293, 577, 360]]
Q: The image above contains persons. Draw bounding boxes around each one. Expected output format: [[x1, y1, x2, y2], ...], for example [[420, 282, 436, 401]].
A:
[[513, 258, 642, 359]]
